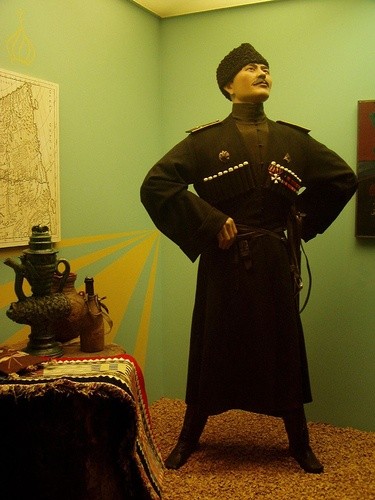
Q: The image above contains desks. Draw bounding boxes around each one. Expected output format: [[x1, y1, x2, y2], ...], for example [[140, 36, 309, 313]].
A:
[[0, 335, 126, 500]]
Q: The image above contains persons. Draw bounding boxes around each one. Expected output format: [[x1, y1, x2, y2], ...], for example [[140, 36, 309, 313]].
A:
[[139, 42, 360, 475]]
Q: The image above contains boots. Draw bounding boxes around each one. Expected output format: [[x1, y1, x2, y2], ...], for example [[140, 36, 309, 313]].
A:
[[165, 409, 208, 469], [280, 408, 323, 473]]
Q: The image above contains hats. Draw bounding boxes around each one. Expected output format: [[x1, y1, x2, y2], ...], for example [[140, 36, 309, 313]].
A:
[[216, 43, 269, 99]]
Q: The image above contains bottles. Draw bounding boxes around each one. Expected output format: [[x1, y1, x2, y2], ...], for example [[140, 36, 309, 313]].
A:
[[80, 277, 104, 352]]
